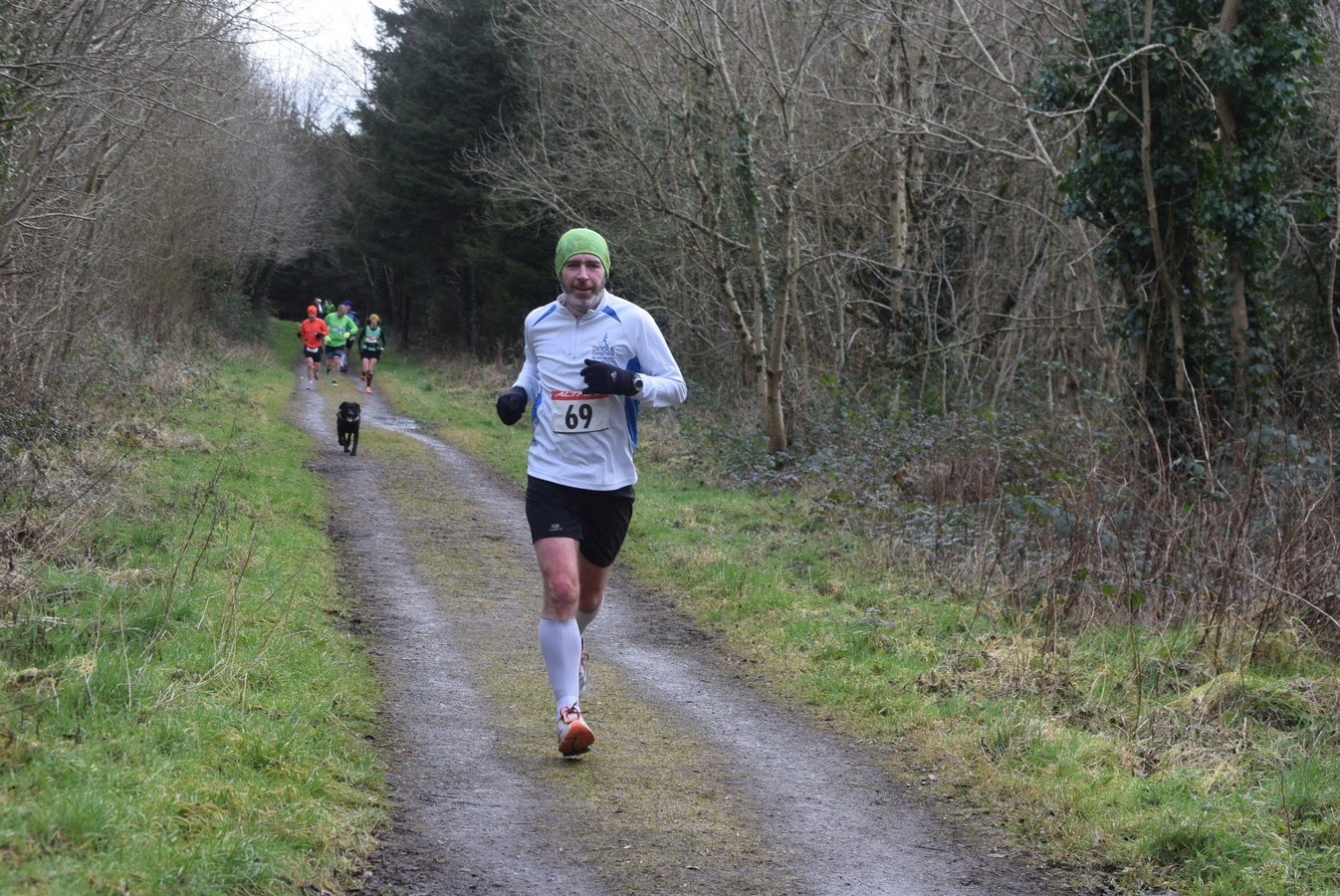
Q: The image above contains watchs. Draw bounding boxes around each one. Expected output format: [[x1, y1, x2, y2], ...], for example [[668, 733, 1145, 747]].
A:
[[627, 370, 643, 398]]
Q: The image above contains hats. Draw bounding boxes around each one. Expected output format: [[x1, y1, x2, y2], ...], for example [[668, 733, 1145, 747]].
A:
[[370, 314, 380, 322], [343, 299, 354, 307], [307, 305, 317, 314], [555, 228, 610, 280]]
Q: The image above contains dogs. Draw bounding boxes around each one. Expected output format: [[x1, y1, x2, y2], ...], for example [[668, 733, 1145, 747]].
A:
[[336, 402, 361, 456]]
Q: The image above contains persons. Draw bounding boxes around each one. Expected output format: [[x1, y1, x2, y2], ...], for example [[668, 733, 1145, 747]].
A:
[[358, 314, 387, 393], [339, 301, 363, 373], [310, 297, 337, 354], [297, 305, 329, 390], [494, 226, 689, 757], [323, 304, 359, 387]]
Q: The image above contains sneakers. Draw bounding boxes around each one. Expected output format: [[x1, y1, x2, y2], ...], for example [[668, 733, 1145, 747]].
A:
[[578, 638, 589, 697], [331, 380, 338, 387], [557, 713, 595, 757], [361, 373, 368, 382], [365, 386, 372, 393]]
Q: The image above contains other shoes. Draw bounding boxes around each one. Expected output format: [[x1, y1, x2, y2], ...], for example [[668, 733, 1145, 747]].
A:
[[325, 366, 331, 374], [305, 381, 313, 390], [340, 365, 348, 374], [313, 370, 319, 379]]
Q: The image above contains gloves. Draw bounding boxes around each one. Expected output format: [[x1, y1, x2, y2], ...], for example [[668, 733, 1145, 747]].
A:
[[580, 359, 635, 396], [315, 332, 323, 338], [324, 336, 334, 345], [343, 331, 351, 339], [297, 332, 301, 338], [496, 386, 527, 427], [367, 346, 376, 351], [349, 335, 356, 341], [377, 347, 384, 351]]
